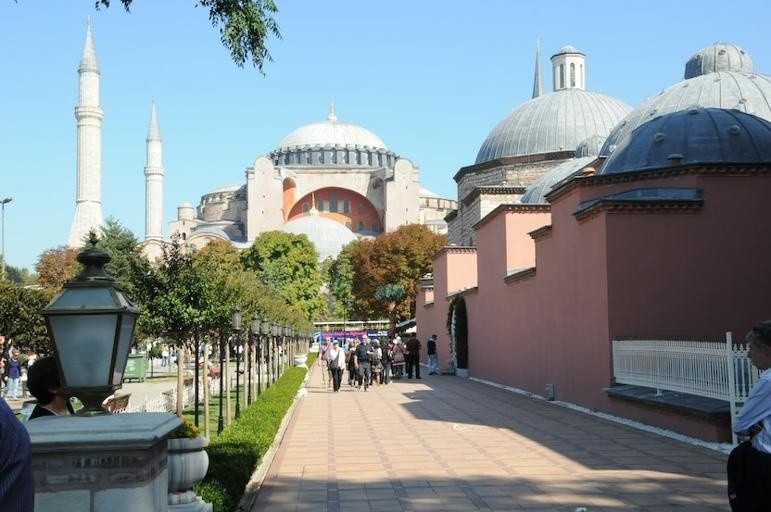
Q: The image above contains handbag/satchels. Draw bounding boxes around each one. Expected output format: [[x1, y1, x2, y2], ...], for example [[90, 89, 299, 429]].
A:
[[728, 436, 753, 512], [328, 359, 339, 371]]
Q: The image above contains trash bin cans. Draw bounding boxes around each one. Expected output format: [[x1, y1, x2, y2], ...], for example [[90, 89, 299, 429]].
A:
[[123, 353, 149, 383]]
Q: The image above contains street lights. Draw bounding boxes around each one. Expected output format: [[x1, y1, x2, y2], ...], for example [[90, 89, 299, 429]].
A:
[[220, 305, 295, 416], [0, 198, 12, 279]]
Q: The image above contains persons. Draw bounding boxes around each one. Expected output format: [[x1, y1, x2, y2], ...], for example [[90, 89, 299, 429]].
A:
[[726, 318, 771, 512], [0, 332, 76, 511], [160, 339, 244, 368], [317, 331, 441, 391]]
